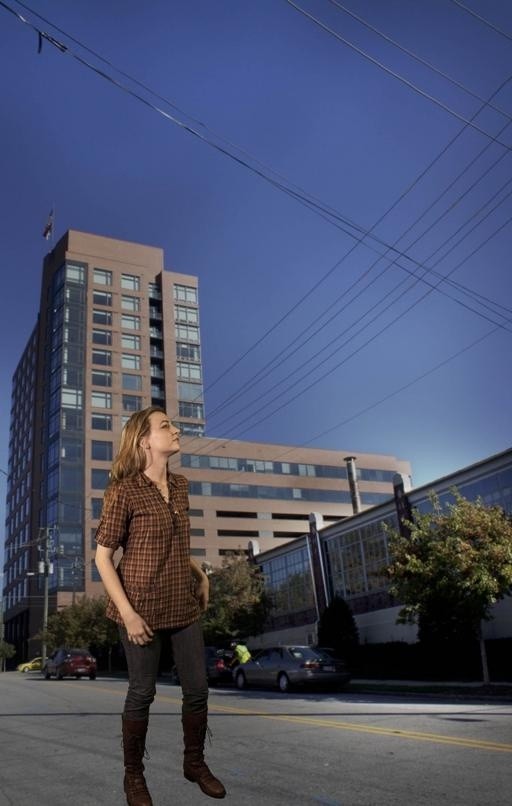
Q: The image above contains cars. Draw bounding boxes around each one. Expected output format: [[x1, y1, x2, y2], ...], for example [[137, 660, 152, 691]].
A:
[[233, 644, 344, 692], [18, 656, 49, 673], [43, 647, 97, 680], [172, 647, 239, 687]]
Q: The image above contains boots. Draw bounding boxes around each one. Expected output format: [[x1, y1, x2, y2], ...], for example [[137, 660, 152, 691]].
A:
[[121, 713, 153, 806], [181, 715, 226, 799]]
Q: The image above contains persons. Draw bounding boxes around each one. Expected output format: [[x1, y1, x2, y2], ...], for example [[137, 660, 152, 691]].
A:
[[229, 641, 251, 667], [91, 404, 228, 806]]
